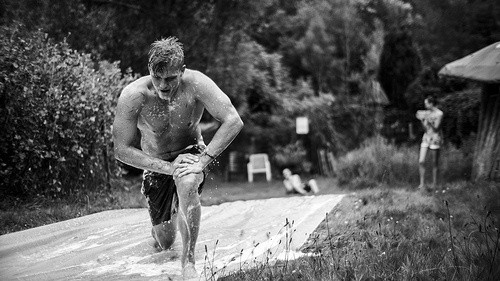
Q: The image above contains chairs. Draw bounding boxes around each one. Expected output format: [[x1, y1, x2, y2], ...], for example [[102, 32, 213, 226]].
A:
[[246, 154, 272, 183], [223, 150, 247, 181]]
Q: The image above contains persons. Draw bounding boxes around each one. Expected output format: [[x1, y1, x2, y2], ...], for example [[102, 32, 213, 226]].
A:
[[283, 169, 319, 200], [416, 98, 444, 192], [110, 39, 245, 281]]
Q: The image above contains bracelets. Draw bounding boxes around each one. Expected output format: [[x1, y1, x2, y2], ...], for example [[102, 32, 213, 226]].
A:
[[203, 151, 215, 163]]
